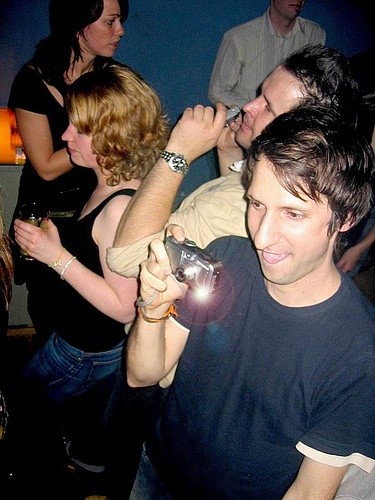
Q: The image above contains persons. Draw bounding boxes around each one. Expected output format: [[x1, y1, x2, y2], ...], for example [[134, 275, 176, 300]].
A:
[[13, 64, 171, 405], [8, 0, 130, 334], [207, 0, 326, 109], [333, 126, 375, 273], [105, 43, 360, 279], [126, 103, 375, 500]]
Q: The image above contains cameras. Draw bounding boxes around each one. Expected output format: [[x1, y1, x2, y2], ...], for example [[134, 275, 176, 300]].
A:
[[225, 105, 240, 128], [165, 236, 222, 295]]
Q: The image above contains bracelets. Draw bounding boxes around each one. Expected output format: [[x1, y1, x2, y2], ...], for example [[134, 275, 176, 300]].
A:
[[48, 247, 65, 269], [137, 304, 178, 324], [60, 256, 77, 280]]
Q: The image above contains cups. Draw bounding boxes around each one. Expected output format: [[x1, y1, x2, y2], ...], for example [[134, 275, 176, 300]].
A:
[[18, 203, 51, 261]]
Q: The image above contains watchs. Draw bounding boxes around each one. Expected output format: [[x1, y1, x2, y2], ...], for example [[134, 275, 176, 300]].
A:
[[159, 150, 189, 174]]
